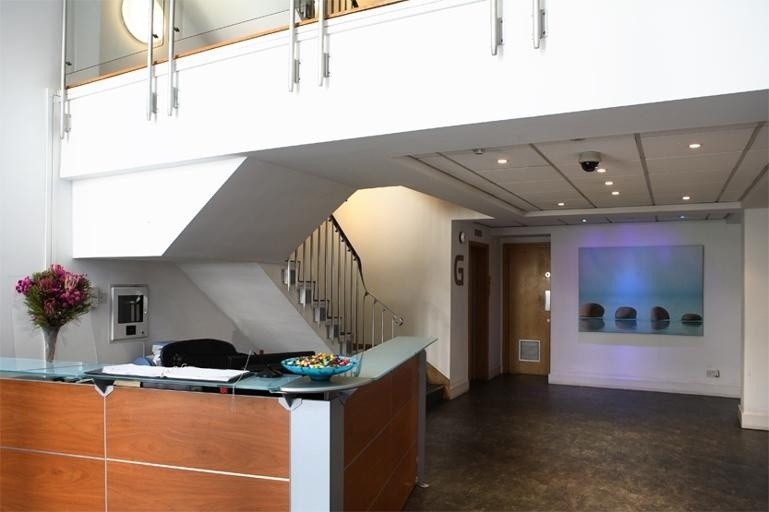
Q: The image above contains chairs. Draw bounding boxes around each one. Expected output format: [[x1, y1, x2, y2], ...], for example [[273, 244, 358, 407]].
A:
[[161, 338, 249, 370]]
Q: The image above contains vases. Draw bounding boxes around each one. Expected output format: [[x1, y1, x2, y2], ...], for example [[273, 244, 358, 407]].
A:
[[42, 327, 60, 363]]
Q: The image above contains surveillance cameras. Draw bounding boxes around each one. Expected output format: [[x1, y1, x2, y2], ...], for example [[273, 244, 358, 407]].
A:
[[579, 150, 600, 172]]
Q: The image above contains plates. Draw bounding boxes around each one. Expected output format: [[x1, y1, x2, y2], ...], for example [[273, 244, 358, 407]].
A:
[[281, 356, 358, 381]]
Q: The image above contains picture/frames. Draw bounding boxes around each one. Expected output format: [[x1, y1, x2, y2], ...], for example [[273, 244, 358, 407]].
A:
[[519, 340, 540, 362]]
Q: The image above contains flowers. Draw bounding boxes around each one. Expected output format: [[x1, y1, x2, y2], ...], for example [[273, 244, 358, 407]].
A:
[[16, 264, 99, 331]]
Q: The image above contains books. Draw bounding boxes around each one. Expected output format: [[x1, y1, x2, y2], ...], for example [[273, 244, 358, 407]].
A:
[[84, 362, 256, 384]]
